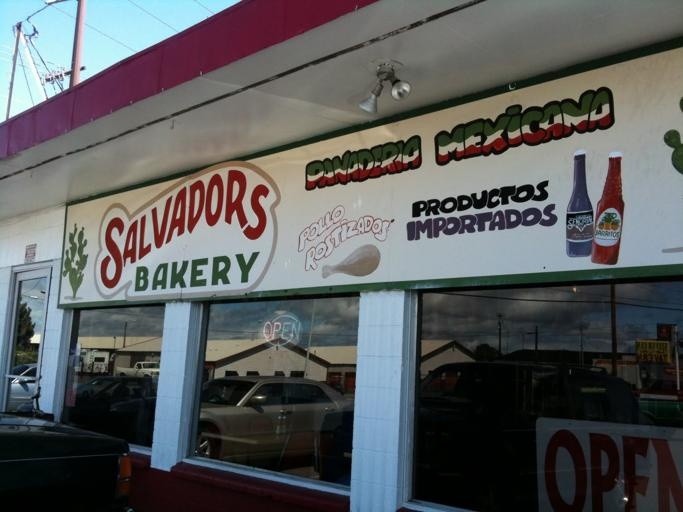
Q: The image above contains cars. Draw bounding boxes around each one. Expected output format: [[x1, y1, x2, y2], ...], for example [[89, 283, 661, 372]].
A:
[[315, 350, 641, 503], [188, 371, 352, 474], [6, 362, 159, 438]]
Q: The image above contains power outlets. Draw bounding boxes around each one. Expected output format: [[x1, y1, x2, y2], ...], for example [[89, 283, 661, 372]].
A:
[[0, 414, 135, 511], [116, 360, 160, 381]]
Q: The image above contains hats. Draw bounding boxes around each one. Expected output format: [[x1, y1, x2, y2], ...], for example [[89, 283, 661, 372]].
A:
[[6, 362, 159, 438], [188, 371, 352, 474]]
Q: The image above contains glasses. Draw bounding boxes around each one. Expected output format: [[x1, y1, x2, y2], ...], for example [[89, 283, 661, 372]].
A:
[[359, 64, 412, 116]]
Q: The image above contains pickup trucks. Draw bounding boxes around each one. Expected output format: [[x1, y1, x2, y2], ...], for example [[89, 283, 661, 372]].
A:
[[116, 360, 160, 381], [0, 414, 135, 511]]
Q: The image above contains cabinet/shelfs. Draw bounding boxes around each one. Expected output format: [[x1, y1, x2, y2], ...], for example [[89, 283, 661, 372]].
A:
[[564, 149, 628, 266]]
